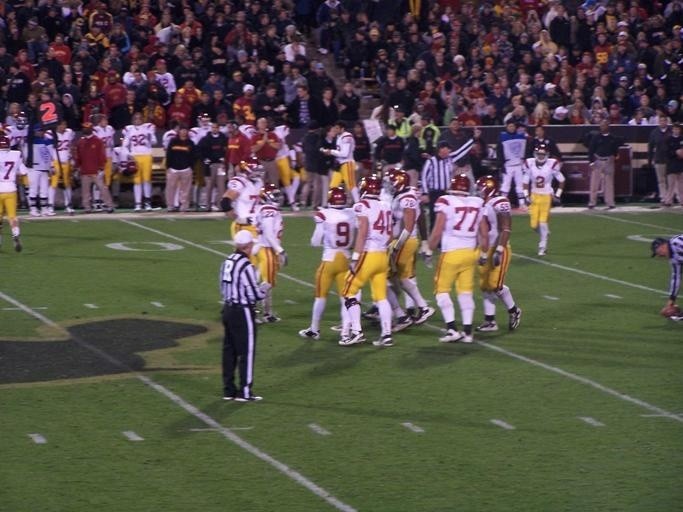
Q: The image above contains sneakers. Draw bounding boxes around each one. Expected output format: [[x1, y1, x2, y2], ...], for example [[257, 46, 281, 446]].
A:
[[223, 391, 262, 403], [28, 203, 222, 218], [458, 329, 474, 344], [262, 313, 281, 324], [438, 328, 460, 342], [518, 197, 615, 257], [330, 300, 436, 347], [289, 202, 321, 210], [12, 233, 22, 252], [508, 306, 522, 332], [477, 321, 501, 331], [661, 200, 682, 206], [298, 326, 320, 340]]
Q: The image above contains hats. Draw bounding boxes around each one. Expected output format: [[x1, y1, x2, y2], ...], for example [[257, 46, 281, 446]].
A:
[[234, 230, 259, 245], [650, 237, 665, 258], [437, 140, 455, 149], [14, 17, 680, 127]]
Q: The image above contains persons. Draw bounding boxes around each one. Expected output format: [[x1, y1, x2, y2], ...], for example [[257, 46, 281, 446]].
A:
[[217, 232, 270, 402], [648, 235, 683, 317]]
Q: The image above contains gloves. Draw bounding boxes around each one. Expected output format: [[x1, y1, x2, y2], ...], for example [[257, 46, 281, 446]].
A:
[[479, 252, 487, 266], [344, 237, 433, 276], [492, 250, 503, 266], [278, 250, 289, 267]]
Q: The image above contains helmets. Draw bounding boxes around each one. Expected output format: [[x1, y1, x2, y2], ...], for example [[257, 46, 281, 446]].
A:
[[241, 159, 266, 182], [0, 128, 12, 149], [474, 174, 499, 198], [326, 166, 411, 207], [534, 143, 550, 163], [446, 173, 470, 195], [260, 182, 284, 207], [197, 115, 212, 126]]
